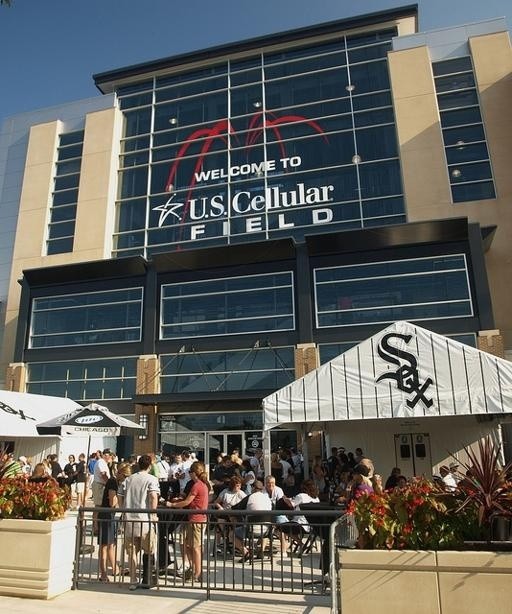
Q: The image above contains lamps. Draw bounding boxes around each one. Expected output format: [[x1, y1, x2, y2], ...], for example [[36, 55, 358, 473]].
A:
[[307, 430, 313, 437], [139, 412, 149, 440]]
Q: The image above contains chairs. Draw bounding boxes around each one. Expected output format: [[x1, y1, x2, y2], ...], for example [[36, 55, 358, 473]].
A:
[[213, 505, 335, 592]]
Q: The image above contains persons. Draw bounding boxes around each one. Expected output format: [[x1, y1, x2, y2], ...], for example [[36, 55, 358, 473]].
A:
[[1, 442, 474, 589]]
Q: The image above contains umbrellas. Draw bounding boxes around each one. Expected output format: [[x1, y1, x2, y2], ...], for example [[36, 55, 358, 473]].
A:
[[36, 402, 146, 506]]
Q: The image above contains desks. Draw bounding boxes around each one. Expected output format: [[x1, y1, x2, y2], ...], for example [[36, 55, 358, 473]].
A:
[[154, 505, 190, 570]]
